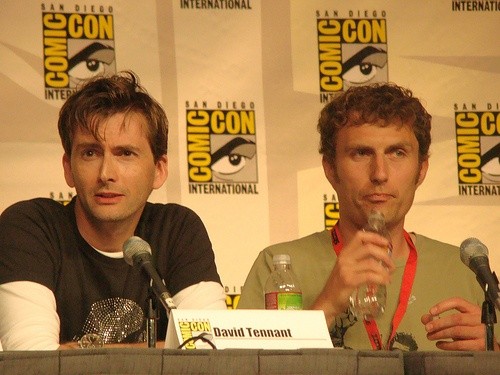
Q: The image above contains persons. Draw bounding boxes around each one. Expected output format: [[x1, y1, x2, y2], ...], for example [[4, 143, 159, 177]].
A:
[[0, 71, 231, 350], [238, 83, 499, 353]]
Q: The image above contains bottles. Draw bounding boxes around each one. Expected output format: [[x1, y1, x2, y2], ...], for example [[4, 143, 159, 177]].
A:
[[264, 254, 303, 310], [348, 210, 392, 321]]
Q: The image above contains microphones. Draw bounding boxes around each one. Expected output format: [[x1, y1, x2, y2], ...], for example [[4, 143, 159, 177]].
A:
[[459, 238, 500, 310], [122, 236, 177, 309]]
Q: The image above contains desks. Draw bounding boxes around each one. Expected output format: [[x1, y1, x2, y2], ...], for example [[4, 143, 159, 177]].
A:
[[0, 347, 405, 375], [405, 350, 500, 375]]
